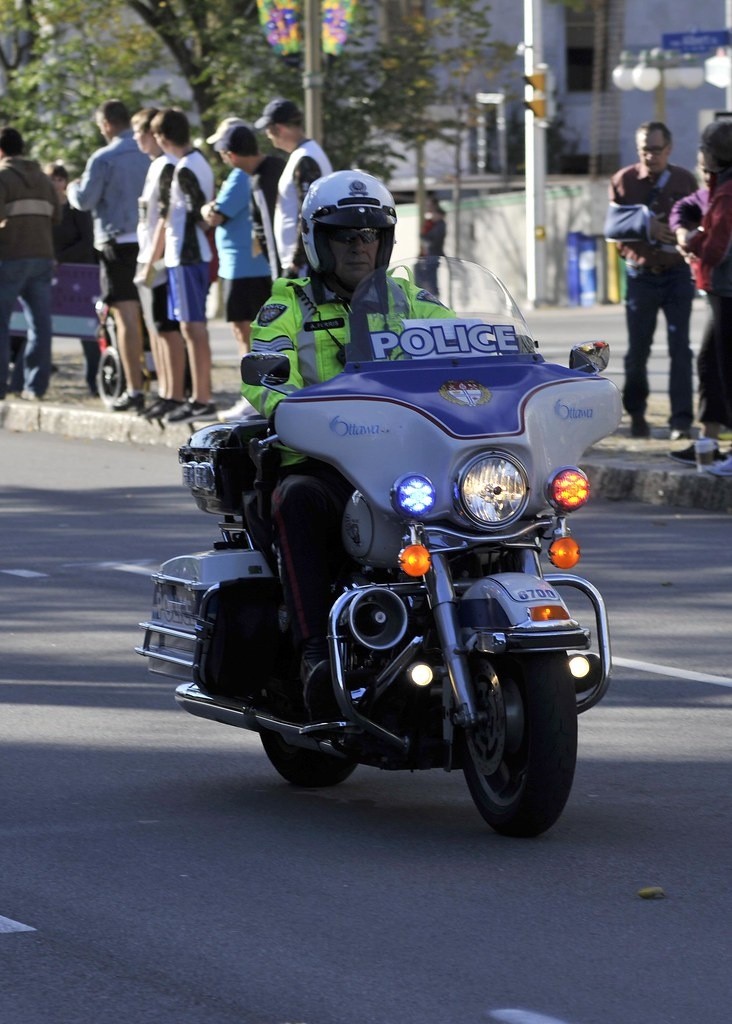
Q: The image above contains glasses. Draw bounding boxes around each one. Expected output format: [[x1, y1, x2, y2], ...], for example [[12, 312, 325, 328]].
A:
[[638, 140, 669, 155], [327, 227, 381, 243]]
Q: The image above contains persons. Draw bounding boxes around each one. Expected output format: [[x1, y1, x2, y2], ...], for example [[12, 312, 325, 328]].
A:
[[609, 121, 732, 437], [0, 127, 108, 400], [241, 171, 461, 712], [199, 101, 332, 420], [67, 100, 214, 425], [415, 200, 446, 298]]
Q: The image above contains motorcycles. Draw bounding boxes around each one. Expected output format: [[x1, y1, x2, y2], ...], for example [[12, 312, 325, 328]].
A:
[[133, 260, 622, 839]]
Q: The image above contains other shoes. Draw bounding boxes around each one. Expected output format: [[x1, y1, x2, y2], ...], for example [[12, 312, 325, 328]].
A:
[[20, 390, 43, 402], [301, 648, 344, 725], [145, 399, 184, 420], [670, 426, 691, 440], [631, 418, 650, 438], [113, 390, 144, 411]]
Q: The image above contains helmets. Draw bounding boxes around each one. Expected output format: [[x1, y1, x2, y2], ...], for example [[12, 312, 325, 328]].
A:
[[299, 170, 395, 269]]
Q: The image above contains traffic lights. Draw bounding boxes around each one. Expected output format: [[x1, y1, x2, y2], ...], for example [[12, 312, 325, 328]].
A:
[[520, 73, 551, 115]]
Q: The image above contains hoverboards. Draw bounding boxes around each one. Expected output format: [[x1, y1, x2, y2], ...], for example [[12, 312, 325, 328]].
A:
[[95, 298, 195, 412]]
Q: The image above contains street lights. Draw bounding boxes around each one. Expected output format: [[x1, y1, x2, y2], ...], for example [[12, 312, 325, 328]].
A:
[[611, 41, 706, 122]]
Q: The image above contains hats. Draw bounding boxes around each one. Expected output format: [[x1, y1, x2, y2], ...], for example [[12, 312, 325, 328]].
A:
[[215, 125, 259, 155], [254, 98, 298, 130], [206, 116, 253, 145]]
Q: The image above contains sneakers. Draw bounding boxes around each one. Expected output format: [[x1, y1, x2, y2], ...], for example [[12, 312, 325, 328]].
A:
[[668, 443, 720, 467], [165, 397, 217, 424], [706, 455, 732, 475]]
